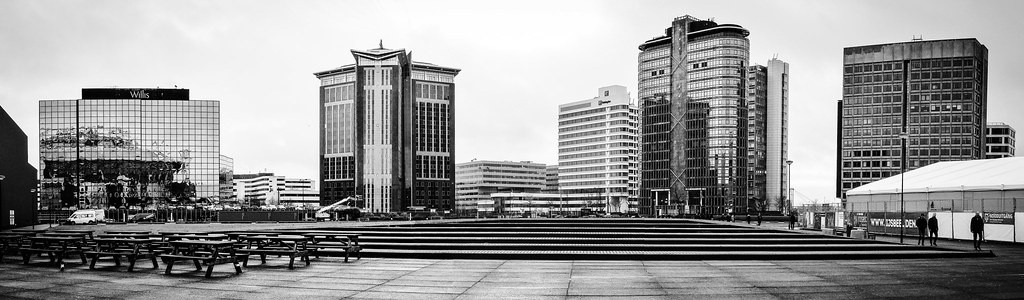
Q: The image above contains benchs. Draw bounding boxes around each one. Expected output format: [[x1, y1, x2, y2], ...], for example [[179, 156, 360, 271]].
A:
[[0, 234, 368, 262]]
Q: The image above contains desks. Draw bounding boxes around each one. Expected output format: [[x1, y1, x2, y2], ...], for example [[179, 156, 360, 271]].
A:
[[11, 228, 49, 248], [86, 237, 161, 273], [300, 233, 361, 263], [238, 235, 310, 270], [161, 239, 247, 279], [22, 236, 87, 268], [164, 235, 229, 265], [52, 229, 96, 251], [96, 233, 154, 261]]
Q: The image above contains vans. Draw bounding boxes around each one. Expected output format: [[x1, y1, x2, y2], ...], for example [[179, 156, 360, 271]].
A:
[[131, 212, 156, 223], [67, 209, 107, 226]]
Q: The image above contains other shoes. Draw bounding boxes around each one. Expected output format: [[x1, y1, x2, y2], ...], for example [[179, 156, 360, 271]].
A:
[[977, 245, 981, 250], [931, 244, 932, 246], [974, 249, 977, 250], [933, 242, 937, 245]]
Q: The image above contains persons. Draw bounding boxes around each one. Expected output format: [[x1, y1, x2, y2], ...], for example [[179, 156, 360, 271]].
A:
[[747, 216, 751, 224], [720, 213, 731, 222], [756, 213, 763, 226], [846, 224, 852, 237], [928, 212, 939, 246], [790, 213, 796, 230], [916, 213, 927, 246], [970, 211, 984, 250], [661, 212, 712, 220]]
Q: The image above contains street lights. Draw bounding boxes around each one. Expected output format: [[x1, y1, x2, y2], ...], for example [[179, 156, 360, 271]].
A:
[[786, 160, 793, 231], [899, 132, 909, 243]]
